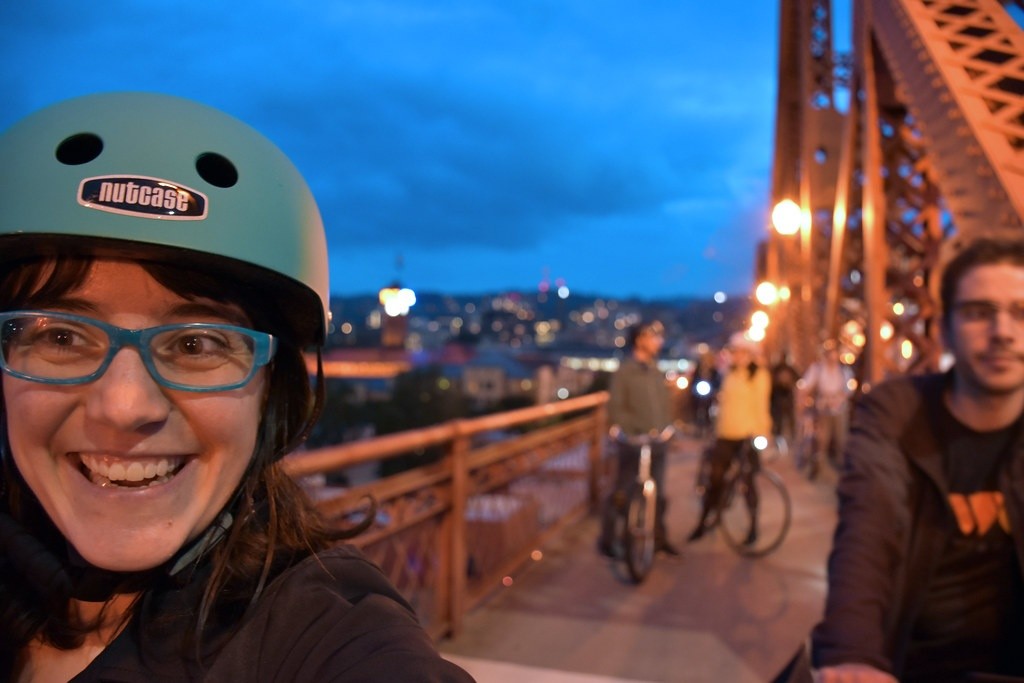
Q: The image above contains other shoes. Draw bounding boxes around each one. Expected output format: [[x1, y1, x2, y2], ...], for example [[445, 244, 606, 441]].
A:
[[658, 543, 677, 556], [597, 541, 618, 558]]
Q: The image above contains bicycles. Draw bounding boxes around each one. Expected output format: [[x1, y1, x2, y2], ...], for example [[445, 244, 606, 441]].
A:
[[698, 416, 793, 557], [605, 424, 677, 583]]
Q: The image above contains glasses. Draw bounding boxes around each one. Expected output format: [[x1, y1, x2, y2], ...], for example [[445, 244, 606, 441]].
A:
[[0, 310, 277, 392]]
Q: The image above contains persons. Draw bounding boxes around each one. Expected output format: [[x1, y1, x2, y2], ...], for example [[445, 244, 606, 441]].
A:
[[0, 94, 479, 683], [691, 346, 856, 481], [599, 320, 680, 558], [690, 332, 771, 543], [811, 236, 1024, 683]]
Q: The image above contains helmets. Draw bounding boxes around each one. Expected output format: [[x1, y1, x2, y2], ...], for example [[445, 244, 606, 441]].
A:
[[0, 92, 330, 348]]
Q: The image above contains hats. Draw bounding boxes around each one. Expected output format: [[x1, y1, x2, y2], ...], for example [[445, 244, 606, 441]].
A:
[[730, 331, 761, 355]]
